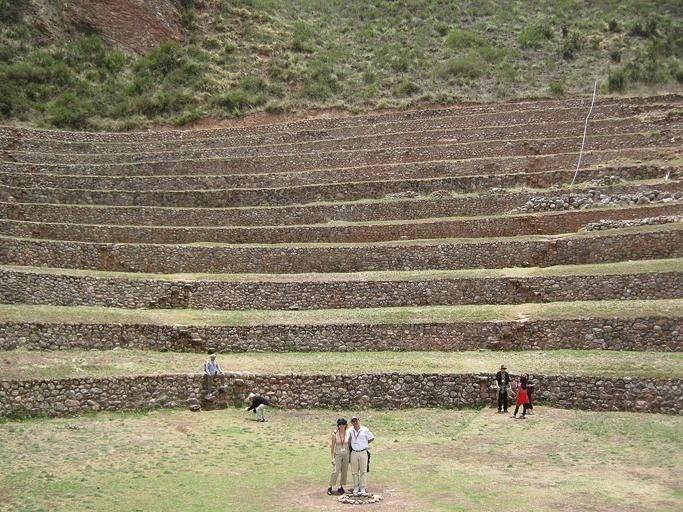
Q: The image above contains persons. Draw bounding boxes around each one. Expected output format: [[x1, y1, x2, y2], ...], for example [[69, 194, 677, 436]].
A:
[[245, 393, 270, 422], [496, 365, 536, 418], [205, 354, 226, 397], [327, 418, 374, 495]]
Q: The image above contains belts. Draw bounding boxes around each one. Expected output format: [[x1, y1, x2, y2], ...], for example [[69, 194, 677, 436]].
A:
[[352, 449, 366, 452]]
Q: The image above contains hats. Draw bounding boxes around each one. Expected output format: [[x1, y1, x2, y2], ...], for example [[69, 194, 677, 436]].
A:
[[351, 416, 359, 421], [337, 418, 347, 424], [248, 393, 255, 398], [501, 365, 507, 369]]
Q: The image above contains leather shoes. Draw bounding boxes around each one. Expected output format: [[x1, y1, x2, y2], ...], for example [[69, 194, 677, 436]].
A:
[[338, 487, 344, 494], [328, 489, 332, 495]]
